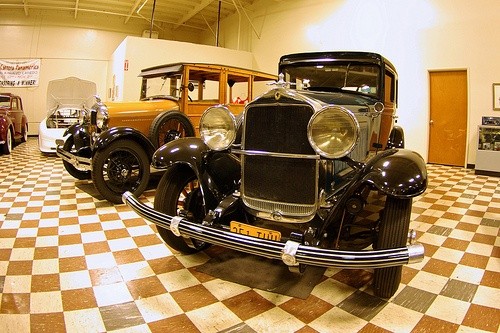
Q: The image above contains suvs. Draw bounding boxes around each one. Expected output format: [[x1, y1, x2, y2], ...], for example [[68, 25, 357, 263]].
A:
[[54, 60, 280, 205], [119, 49, 431, 301]]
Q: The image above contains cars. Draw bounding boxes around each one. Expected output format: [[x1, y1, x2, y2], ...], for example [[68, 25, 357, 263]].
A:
[[38, 107, 93, 156], [0, 92, 29, 153]]
